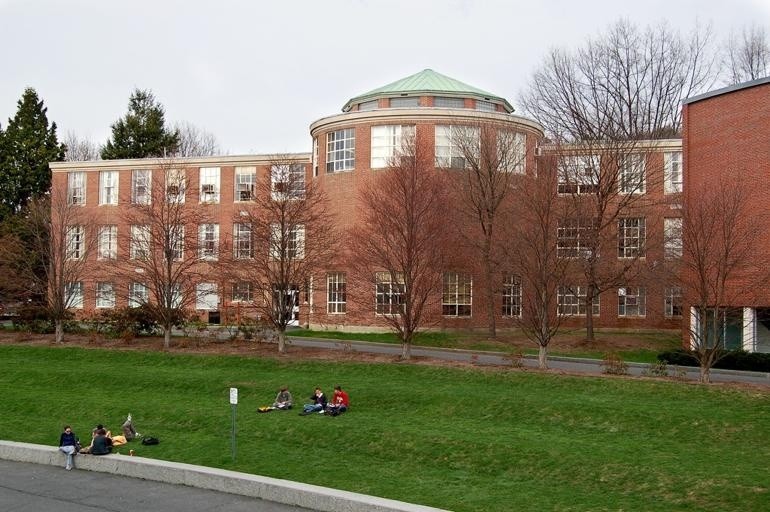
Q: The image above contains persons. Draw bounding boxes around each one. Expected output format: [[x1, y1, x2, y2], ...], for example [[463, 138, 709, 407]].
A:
[[59, 425, 78, 470], [302, 386, 328, 412], [325, 385, 349, 416], [90, 424, 114, 455], [111, 413, 138, 447], [273, 385, 292, 410]]
